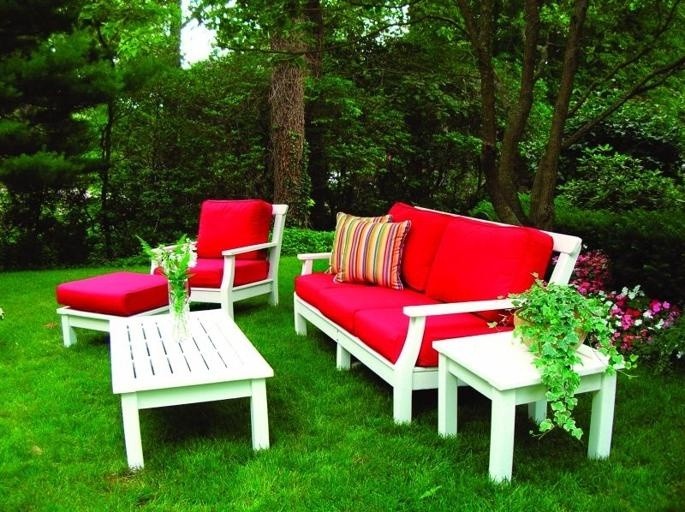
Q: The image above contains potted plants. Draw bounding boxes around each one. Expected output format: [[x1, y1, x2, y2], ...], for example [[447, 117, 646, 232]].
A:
[[485, 273, 639, 442]]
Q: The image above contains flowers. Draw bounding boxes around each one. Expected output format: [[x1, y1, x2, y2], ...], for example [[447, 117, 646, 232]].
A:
[[131, 231, 199, 329]]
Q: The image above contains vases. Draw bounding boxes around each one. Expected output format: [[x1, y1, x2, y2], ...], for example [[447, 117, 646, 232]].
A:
[[167, 280, 189, 339]]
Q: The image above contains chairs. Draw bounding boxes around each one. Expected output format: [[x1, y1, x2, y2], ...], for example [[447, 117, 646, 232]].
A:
[[148, 203, 289, 320]]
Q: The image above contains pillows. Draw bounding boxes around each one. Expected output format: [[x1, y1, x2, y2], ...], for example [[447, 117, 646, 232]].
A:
[[322, 210, 393, 274], [333, 214, 412, 291], [197, 199, 272, 260], [385, 201, 455, 291], [425, 221, 553, 322]]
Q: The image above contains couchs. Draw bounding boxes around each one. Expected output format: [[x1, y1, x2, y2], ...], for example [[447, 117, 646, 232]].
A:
[[291, 205, 583, 427]]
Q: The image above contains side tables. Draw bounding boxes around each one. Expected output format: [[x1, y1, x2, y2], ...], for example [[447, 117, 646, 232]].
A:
[[431, 331, 624, 485]]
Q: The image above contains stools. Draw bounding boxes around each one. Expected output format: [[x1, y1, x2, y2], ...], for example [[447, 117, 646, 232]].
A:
[[55, 272, 190, 347]]
